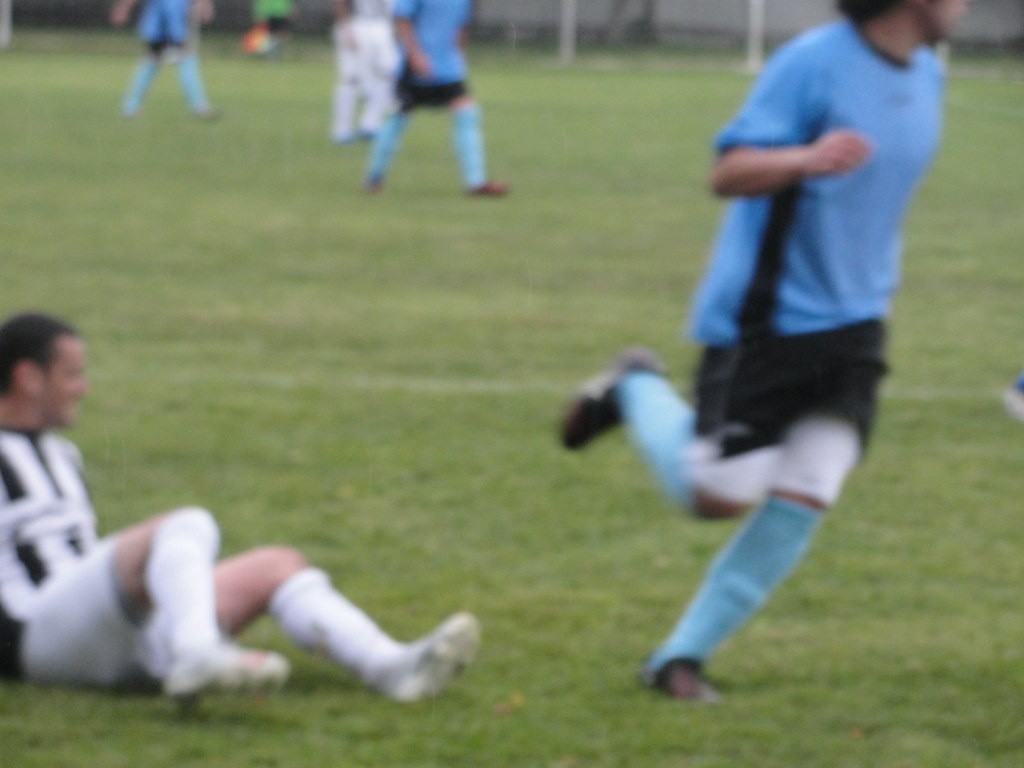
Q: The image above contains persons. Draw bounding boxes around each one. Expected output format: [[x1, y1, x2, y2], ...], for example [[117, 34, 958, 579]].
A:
[[110, 1, 221, 119], [250, 0, 301, 63], [331, 0, 403, 150], [362, 0, 514, 200], [559, 1, 970, 701], [0, 313, 484, 707]]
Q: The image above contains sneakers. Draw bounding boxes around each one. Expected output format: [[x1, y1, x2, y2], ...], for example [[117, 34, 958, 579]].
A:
[[167, 639, 291, 714], [643, 660, 720, 705], [381, 614, 485, 705], [563, 347, 665, 449]]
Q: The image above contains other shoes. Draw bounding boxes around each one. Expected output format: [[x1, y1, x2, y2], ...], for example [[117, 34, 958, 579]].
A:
[[360, 179, 381, 196], [188, 109, 218, 118], [471, 180, 506, 197], [332, 126, 375, 150], [120, 106, 143, 118]]
